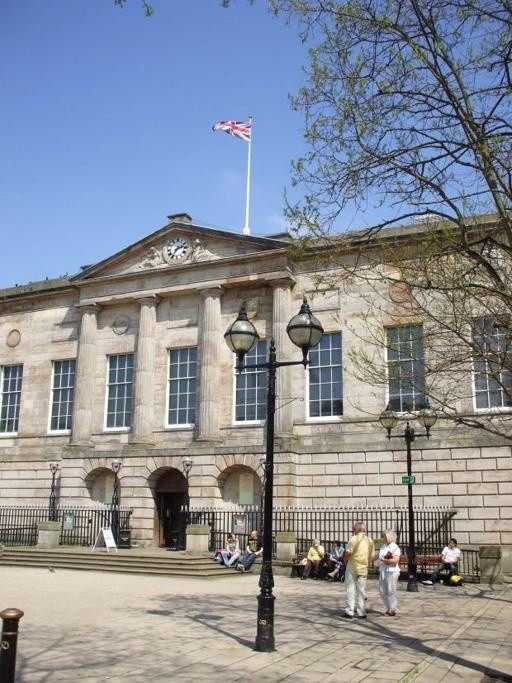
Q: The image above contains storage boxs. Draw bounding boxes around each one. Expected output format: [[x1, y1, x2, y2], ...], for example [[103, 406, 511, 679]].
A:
[[290, 551, 333, 578], [398, 552, 462, 585]]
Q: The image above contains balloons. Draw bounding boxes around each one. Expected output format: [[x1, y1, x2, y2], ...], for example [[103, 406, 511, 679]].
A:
[[163, 233, 193, 264]]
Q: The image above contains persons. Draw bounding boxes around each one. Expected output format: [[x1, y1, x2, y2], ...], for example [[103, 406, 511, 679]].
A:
[[300, 538, 325, 580], [339, 522, 375, 621], [236, 530, 264, 572], [421, 538, 461, 585], [372, 529, 402, 616], [220, 533, 241, 569], [327, 540, 345, 578]]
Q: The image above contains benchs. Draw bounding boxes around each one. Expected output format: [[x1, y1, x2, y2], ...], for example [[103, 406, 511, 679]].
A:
[[212, 120, 251, 144]]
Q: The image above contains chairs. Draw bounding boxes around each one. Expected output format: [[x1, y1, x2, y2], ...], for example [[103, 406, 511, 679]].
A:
[[300, 573, 339, 582], [237, 563, 245, 573], [421, 579, 434, 585], [355, 614, 368, 620], [338, 614, 354, 621]]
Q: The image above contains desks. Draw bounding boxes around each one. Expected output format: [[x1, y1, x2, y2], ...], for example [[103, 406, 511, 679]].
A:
[[178, 457, 194, 549], [382, 404, 438, 593], [47, 460, 60, 520], [105, 456, 123, 547], [227, 298, 326, 654]]
